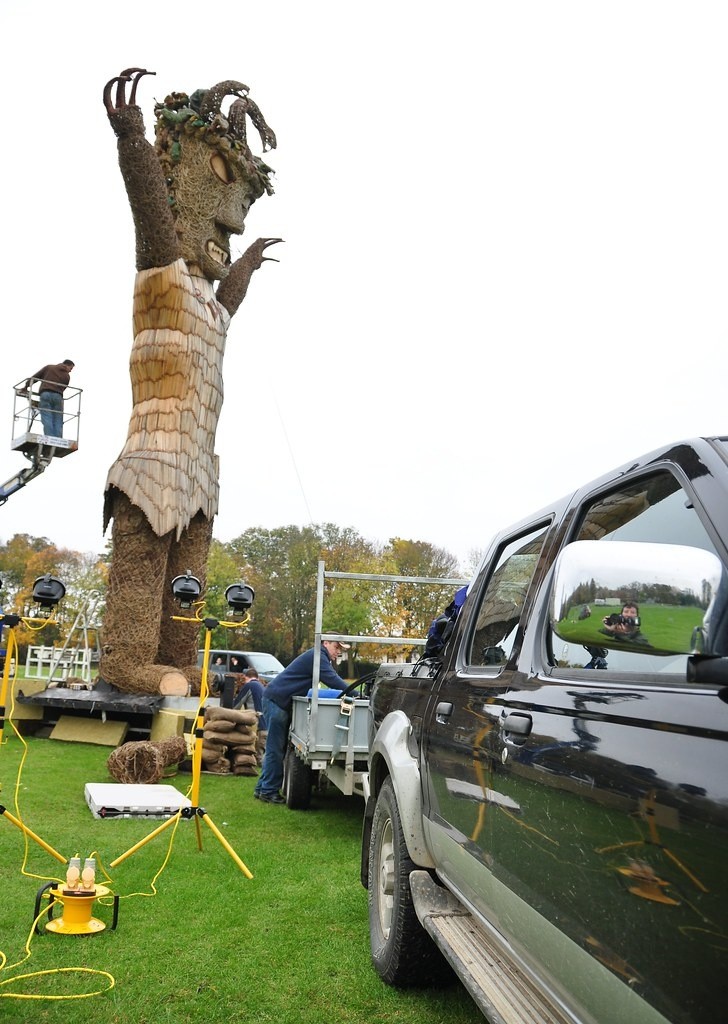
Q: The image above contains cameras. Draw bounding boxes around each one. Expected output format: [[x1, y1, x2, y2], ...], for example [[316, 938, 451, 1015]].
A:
[[602, 613, 642, 627]]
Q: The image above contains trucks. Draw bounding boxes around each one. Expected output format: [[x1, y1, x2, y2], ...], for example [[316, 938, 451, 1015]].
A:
[[280, 561, 473, 809]]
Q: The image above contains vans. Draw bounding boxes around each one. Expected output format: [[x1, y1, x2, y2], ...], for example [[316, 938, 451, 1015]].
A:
[[198, 650, 285, 682]]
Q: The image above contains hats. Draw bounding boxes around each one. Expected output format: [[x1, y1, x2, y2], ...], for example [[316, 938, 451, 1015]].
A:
[[324, 631, 351, 650]]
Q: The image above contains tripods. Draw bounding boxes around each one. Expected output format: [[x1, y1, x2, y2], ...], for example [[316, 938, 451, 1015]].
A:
[[108, 616, 255, 881]]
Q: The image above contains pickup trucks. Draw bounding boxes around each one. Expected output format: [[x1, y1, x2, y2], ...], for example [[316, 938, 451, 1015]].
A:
[[360, 435, 728, 1024]]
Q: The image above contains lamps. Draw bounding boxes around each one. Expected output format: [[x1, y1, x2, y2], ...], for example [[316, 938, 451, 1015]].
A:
[[170, 570, 201, 608], [223, 580, 255, 614], [32, 572, 66, 610]]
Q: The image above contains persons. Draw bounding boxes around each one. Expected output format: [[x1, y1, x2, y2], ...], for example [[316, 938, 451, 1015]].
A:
[[20, 359, 75, 438], [230, 657, 243, 672], [254, 631, 359, 805], [212, 658, 226, 672], [233, 668, 268, 767], [602, 601, 652, 649], [579, 605, 590, 621]]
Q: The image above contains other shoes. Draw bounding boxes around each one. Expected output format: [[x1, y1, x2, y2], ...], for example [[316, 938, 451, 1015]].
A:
[[259, 790, 286, 805], [254, 788, 262, 798]]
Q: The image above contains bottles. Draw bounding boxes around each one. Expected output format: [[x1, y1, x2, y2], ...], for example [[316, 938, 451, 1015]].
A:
[[66, 857, 81, 891], [81, 858, 96, 893]]
